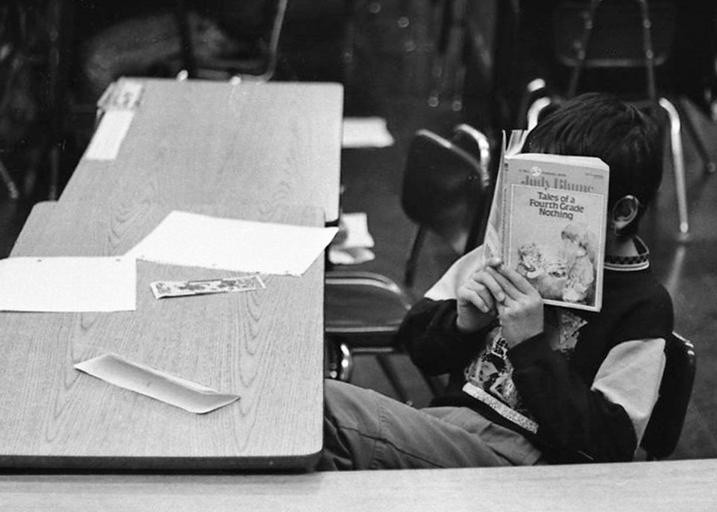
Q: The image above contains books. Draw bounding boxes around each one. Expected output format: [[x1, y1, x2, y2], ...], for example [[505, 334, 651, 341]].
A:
[[483, 129, 610, 313]]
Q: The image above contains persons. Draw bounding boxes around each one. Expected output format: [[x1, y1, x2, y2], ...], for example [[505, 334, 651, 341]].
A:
[[318, 90, 675, 471]]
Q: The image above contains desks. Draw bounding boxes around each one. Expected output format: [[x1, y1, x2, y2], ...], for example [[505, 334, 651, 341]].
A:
[[0, 68, 344, 472]]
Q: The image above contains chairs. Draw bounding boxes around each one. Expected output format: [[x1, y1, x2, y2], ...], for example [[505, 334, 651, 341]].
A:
[[322, 125, 698, 459]]
